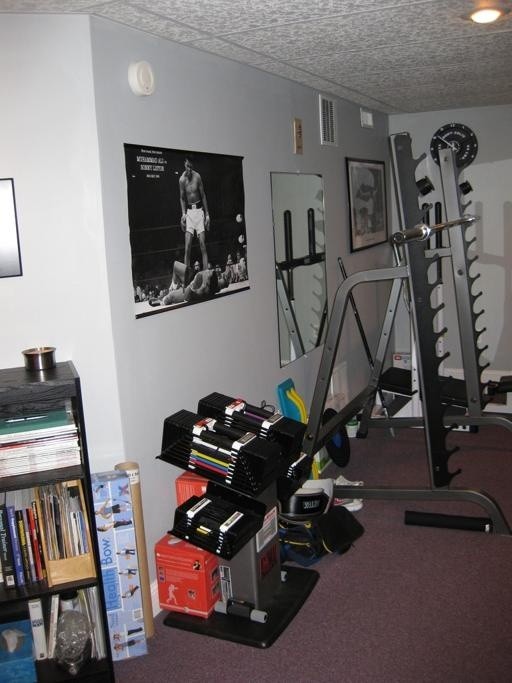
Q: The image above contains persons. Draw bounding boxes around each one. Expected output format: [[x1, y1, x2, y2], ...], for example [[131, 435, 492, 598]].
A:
[[93, 495, 148, 654], [177, 154, 212, 273], [133, 252, 247, 313]]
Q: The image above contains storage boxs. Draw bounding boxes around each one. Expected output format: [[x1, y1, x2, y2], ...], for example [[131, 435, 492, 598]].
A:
[[154, 534, 221, 619], [92, 470, 147, 662], [174, 470, 209, 507]]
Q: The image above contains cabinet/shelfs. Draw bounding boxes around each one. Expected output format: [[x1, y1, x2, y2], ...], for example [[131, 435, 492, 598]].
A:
[[0, 360, 114, 683]]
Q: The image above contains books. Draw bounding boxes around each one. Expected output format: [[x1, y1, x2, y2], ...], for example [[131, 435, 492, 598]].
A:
[[0, 402, 108, 663]]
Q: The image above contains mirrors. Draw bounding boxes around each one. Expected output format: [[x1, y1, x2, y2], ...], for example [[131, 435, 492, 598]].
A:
[[270, 172, 328, 368]]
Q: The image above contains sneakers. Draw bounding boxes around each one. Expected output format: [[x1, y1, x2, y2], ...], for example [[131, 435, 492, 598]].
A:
[[148, 298, 161, 306]]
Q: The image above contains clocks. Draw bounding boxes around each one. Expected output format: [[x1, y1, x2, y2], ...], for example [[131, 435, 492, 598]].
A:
[[430, 122, 478, 169]]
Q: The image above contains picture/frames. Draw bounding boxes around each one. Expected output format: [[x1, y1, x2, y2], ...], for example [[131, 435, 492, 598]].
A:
[[344, 156, 388, 253], [0, 178, 23, 279]]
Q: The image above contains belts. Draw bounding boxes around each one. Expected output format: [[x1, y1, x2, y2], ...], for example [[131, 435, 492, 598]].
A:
[[187, 203, 201, 209]]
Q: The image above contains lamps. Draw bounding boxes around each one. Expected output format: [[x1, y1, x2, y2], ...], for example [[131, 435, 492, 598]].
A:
[[460, 6, 511, 24]]
